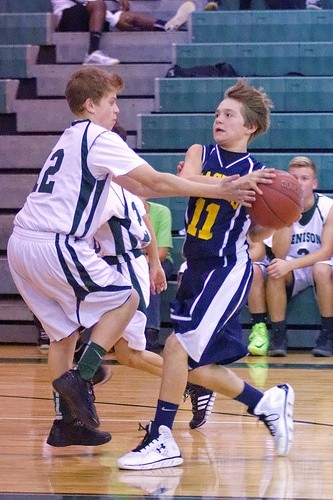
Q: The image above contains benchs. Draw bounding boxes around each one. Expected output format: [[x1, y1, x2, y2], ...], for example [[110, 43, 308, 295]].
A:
[[0, 0, 333, 347]]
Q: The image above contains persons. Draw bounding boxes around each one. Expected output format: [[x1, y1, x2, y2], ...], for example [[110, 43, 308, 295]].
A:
[[51, 0, 332, 355], [72, 123, 217, 428], [115, 79, 293, 469], [8, 68, 257, 449]]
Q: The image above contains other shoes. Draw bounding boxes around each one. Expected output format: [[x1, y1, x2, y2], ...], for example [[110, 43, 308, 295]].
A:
[[145, 329, 159, 353], [267, 329, 288, 356], [82, 50, 120, 66], [165, 1, 196, 32], [52, 390, 63, 416], [37, 329, 50, 348], [310, 324, 333, 357]]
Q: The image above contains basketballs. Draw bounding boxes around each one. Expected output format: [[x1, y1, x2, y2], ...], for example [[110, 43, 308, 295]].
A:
[[245, 169, 304, 228]]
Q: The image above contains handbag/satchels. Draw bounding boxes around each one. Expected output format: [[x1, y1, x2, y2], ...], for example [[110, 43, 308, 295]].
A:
[[166, 62, 237, 78]]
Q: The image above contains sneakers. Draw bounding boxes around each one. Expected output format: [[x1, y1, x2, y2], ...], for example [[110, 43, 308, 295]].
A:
[[248, 322, 269, 355], [185, 429, 220, 492], [247, 382, 295, 456], [262, 456, 293, 500], [184, 382, 216, 429], [116, 420, 183, 470], [51, 366, 100, 428], [116, 467, 184, 500], [46, 419, 112, 447]]
